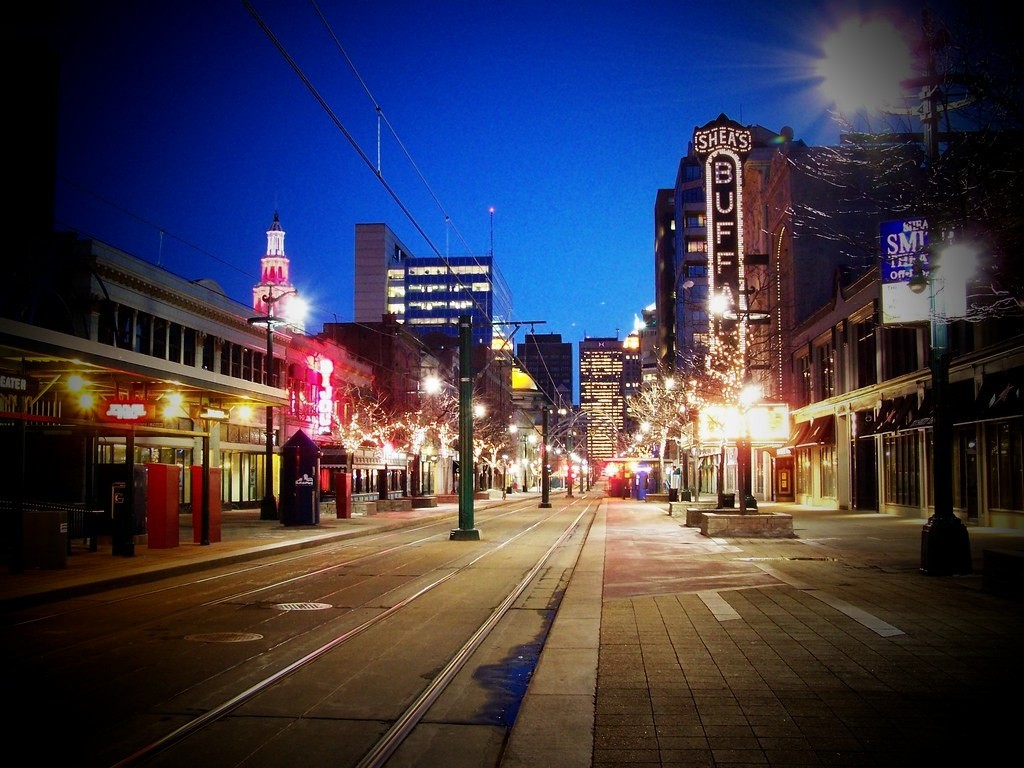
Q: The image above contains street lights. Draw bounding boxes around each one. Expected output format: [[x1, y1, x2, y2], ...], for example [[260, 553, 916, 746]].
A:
[[259, 282, 314, 522], [908, 242, 984, 575]]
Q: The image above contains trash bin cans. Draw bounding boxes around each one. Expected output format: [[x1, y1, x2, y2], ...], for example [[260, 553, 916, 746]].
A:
[[670, 488, 678, 502], [507, 486, 513, 494], [721, 493, 735, 507]]
